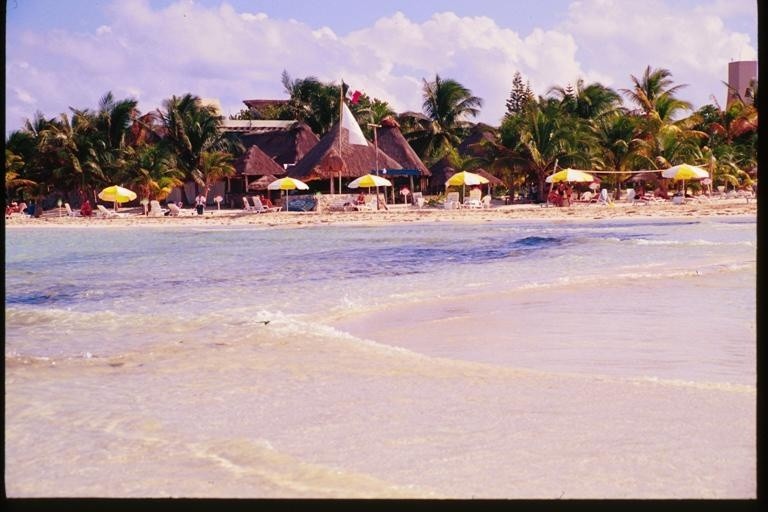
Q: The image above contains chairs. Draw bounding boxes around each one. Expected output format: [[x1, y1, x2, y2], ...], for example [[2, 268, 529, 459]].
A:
[[96, 204, 119, 218], [444, 188, 492, 209], [147, 200, 193, 218], [243, 196, 283, 215], [588, 188, 607, 206], [64, 202, 81, 217], [330, 195, 377, 211]]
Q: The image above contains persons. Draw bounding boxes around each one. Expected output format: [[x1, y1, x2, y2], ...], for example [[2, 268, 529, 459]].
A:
[[195, 192, 205, 215], [701, 181, 709, 195], [684, 184, 695, 199], [353, 191, 366, 207], [634, 185, 643, 200], [5, 198, 42, 218], [671, 188, 683, 198], [555, 182, 566, 196], [505, 182, 538, 204], [610, 187, 617, 203], [481, 194, 491, 209], [578, 189, 593, 202], [549, 188, 562, 206], [565, 187, 572, 206], [77, 189, 88, 207], [177, 200, 184, 209], [80, 199, 92, 218], [750, 182, 757, 204], [260, 194, 273, 209]]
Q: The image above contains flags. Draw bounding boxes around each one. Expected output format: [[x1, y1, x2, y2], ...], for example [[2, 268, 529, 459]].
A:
[[340, 101, 369, 148], [342, 81, 362, 107]]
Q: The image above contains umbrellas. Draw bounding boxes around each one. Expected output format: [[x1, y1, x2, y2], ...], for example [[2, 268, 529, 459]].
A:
[[542, 167, 594, 186], [661, 163, 710, 196], [347, 173, 393, 196], [268, 176, 310, 212], [445, 170, 490, 204], [98, 184, 136, 213]]
